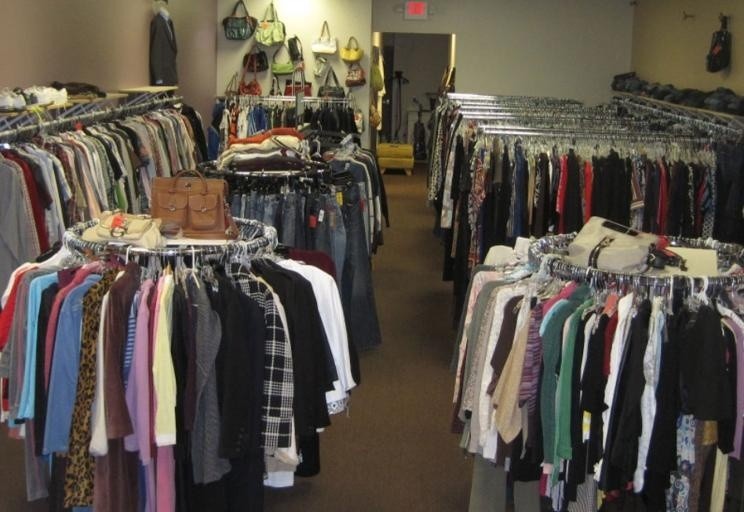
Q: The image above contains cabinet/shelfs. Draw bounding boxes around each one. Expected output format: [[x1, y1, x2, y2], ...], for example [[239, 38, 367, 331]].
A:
[[406, 106, 432, 162]]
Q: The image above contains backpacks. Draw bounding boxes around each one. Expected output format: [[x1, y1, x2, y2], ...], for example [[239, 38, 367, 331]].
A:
[[707, 29, 729, 73]]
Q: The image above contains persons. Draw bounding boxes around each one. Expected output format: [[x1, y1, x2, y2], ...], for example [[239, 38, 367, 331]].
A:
[[149, 4, 180, 97]]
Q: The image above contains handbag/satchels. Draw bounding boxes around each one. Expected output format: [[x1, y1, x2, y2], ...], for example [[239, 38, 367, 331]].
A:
[[81, 212, 165, 250], [611, 72, 744, 114], [150, 168, 238, 240], [370, 47, 383, 92], [369, 101, 380, 127], [223, 0, 366, 97], [567, 215, 685, 274]]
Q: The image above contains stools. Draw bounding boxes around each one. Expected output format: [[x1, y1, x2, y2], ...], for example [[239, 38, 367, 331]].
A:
[[377, 142, 413, 176]]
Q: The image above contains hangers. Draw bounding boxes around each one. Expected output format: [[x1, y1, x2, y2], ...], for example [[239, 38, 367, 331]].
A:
[[474, 228, 744, 340], [213, 91, 357, 119], [0, 212, 338, 301], [2, 92, 187, 178], [196, 131, 375, 196], [437, 93, 744, 168]]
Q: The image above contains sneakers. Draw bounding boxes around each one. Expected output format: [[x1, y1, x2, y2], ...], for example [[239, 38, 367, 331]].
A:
[[0, 86, 68, 112]]
